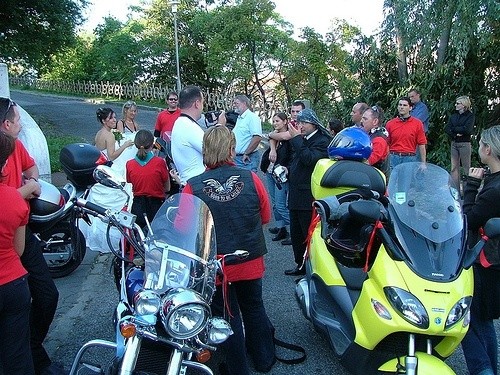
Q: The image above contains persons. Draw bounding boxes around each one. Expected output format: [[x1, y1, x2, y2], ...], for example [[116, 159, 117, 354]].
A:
[[170, 85, 226, 186], [0, 130, 36, 375], [408, 89, 428, 192], [277, 108, 333, 275], [268, 101, 306, 244], [174, 124, 277, 375], [231, 95, 262, 174], [154, 91, 181, 141], [445, 96, 474, 182], [126, 130, 170, 229], [385, 97, 427, 205], [461, 124, 500, 375], [95, 101, 140, 252], [325, 103, 390, 209], [0, 97, 70, 375]]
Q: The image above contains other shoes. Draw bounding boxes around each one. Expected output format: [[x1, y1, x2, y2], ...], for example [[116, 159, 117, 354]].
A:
[[32, 344, 51, 368], [254, 356, 276, 373], [219, 363, 230, 375], [281, 239, 293, 245], [271, 231, 287, 241], [269, 227, 279, 233], [285, 265, 306, 276]]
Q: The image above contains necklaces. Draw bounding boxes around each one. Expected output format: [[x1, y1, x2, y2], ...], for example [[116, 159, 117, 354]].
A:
[[124, 119, 134, 124]]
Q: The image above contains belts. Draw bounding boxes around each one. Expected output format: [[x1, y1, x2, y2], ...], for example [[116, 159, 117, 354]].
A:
[[236, 150, 257, 156], [390, 152, 415, 156]]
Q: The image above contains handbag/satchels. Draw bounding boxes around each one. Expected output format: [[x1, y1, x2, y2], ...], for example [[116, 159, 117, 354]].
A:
[[261, 148, 270, 173]]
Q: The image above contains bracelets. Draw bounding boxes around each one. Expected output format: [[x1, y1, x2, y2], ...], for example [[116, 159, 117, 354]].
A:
[[29, 177, 37, 182]]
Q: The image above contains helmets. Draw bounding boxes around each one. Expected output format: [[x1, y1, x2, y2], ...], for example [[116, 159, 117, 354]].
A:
[[327, 127, 373, 162]]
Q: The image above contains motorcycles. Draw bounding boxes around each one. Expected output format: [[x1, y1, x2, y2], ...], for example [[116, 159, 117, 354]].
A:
[[67, 164, 250, 375], [22, 176, 92, 280], [293, 158, 500, 375], [59, 132, 181, 198]]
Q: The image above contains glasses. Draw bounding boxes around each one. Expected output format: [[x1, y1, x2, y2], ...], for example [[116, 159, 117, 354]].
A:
[[456, 102, 462, 105], [372, 104, 381, 115], [2, 97, 15, 123], [169, 98, 178, 101], [297, 121, 309, 126]]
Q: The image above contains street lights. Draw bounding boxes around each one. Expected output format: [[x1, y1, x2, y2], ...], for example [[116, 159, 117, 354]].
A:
[[168, 2, 183, 96]]
